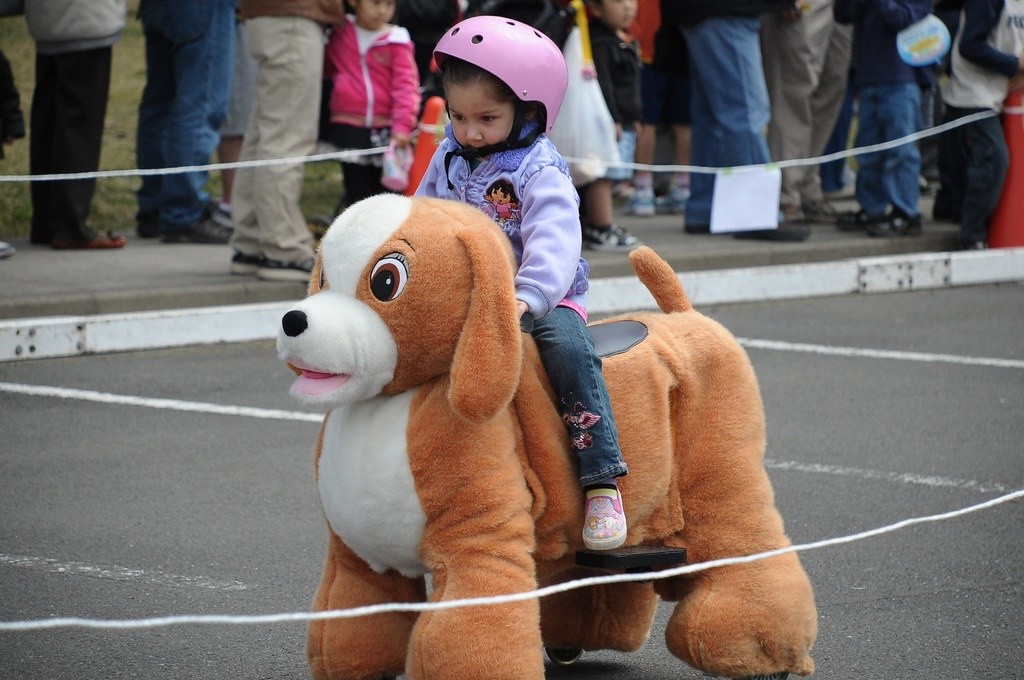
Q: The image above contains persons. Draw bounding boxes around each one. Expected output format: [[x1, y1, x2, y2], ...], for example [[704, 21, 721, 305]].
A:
[[0, 0, 30, 257], [27, 0, 127, 249], [135, 0, 235, 243], [414, 16, 629, 549], [218, 0, 1023, 282]]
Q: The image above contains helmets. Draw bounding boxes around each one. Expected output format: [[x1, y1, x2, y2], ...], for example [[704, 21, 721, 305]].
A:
[[432, 15, 569, 134]]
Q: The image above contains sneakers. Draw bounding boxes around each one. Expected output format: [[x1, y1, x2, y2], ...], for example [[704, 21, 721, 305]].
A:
[[162, 212, 232, 244], [582, 478, 627, 550], [135, 212, 161, 237]]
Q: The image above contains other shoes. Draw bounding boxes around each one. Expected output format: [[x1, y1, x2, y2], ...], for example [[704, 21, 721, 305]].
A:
[[965, 242, 988, 249], [920, 181, 932, 194], [780, 204, 812, 239], [0, 241, 16, 257], [623, 189, 656, 215], [584, 224, 643, 251], [736, 229, 778, 240], [51, 232, 125, 248], [804, 200, 837, 223], [231, 252, 261, 274], [683, 224, 709, 234], [258, 252, 315, 282], [655, 188, 691, 211]]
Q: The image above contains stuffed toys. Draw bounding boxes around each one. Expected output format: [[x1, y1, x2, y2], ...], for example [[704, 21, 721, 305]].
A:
[[278, 193, 817, 680]]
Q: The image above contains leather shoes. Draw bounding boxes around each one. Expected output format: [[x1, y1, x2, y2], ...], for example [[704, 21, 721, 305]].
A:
[[866, 212, 922, 236], [839, 210, 888, 227]]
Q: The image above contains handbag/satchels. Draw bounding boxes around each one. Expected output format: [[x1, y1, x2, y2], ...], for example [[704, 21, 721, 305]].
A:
[[549, 0, 622, 186]]
